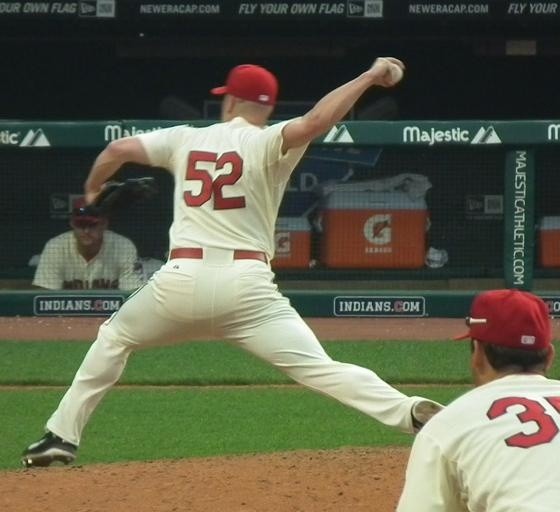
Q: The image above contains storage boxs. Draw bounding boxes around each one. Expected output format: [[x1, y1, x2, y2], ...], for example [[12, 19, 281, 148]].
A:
[[534, 213, 560, 271], [270, 213, 314, 268], [314, 180, 432, 272]]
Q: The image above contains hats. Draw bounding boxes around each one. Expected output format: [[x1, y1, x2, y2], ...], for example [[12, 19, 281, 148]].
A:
[[450, 287, 553, 350], [73, 197, 102, 217], [209, 63, 281, 106]]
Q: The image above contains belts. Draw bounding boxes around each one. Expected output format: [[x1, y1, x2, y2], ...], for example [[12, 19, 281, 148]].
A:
[[169, 247, 268, 263]]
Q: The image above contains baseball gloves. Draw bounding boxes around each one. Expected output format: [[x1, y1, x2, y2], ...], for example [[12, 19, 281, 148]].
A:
[[85, 178, 160, 219]]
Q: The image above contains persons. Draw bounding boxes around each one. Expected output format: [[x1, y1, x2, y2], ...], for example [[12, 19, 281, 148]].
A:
[[390, 287, 559, 511], [22, 53, 446, 465], [31, 194, 146, 291]]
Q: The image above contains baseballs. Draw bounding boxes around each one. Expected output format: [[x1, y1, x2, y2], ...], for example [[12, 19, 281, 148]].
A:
[[386, 63, 403, 85]]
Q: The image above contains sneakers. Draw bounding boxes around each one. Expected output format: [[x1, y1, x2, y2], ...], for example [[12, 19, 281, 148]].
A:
[[409, 398, 443, 434], [19, 432, 78, 470]]
